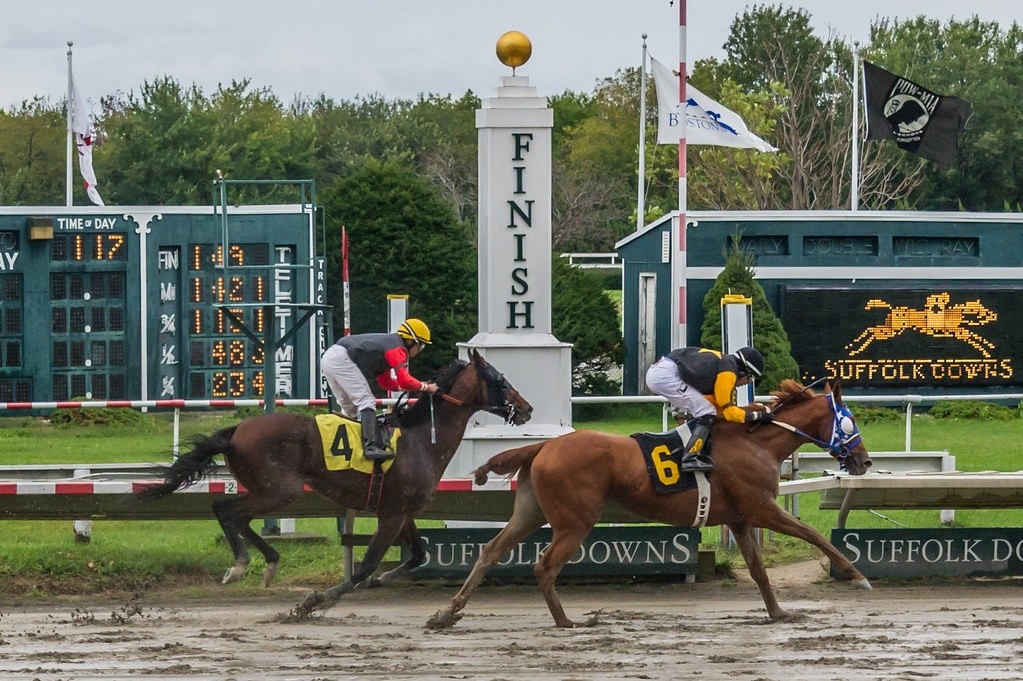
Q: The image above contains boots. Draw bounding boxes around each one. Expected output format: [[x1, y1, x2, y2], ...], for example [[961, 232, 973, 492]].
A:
[[360, 408, 394, 459], [681, 424, 714, 472]]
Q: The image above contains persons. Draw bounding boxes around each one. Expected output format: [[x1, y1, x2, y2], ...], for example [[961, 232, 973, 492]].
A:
[[320, 317, 440, 462], [644, 344, 767, 473]]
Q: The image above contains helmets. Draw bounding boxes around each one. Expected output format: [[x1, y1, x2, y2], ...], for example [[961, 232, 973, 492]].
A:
[[397, 318, 433, 344], [734, 347, 764, 378]]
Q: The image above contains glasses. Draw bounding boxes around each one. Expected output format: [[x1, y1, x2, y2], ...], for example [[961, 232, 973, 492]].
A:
[[739, 350, 756, 381], [402, 321, 426, 352]]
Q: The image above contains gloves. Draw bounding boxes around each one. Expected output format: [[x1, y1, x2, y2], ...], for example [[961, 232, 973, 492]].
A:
[[747, 411, 776, 423]]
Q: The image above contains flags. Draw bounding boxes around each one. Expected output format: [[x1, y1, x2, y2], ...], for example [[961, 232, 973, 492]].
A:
[[862, 59, 973, 170], [649, 56, 781, 153], [71, 76, 106, 206]]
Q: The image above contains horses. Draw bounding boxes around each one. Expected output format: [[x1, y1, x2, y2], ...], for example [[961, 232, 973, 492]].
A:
[[424, 377, 874, 628], [113, 346, 535, 616]]
